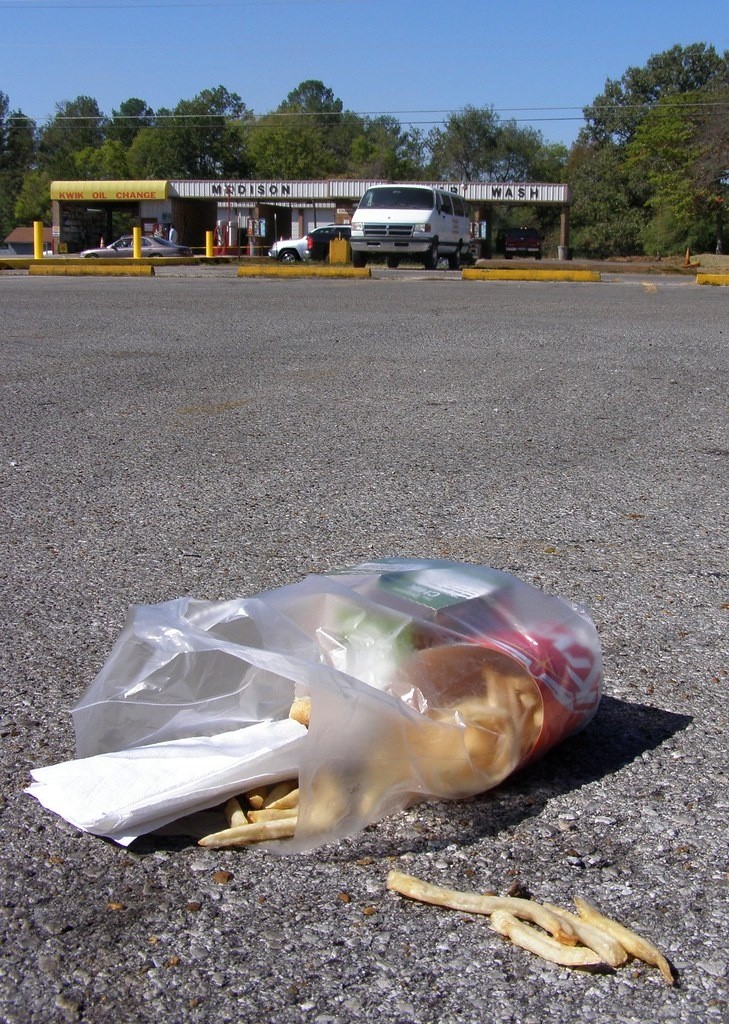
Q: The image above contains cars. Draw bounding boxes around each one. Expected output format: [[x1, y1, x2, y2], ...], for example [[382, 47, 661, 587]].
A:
[[267, 223, 351, 264], [495, 226, 546, 260], [79, 234, 194, 258], [305, 225, 352, 262]]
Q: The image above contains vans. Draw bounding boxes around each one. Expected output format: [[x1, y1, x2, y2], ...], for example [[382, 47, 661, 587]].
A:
[[349, 184, 472, 270]]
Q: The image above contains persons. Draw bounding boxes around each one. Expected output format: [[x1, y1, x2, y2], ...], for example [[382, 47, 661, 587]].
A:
[[169, 225, 177, 245]]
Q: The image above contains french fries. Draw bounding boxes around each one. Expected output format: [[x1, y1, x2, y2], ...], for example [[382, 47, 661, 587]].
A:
[[386, 871, 675, 984], [195, 665, 540, 847]]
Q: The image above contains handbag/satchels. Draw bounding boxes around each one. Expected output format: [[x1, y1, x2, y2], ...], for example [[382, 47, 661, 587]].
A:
[[74, 559, 604, 857]]
